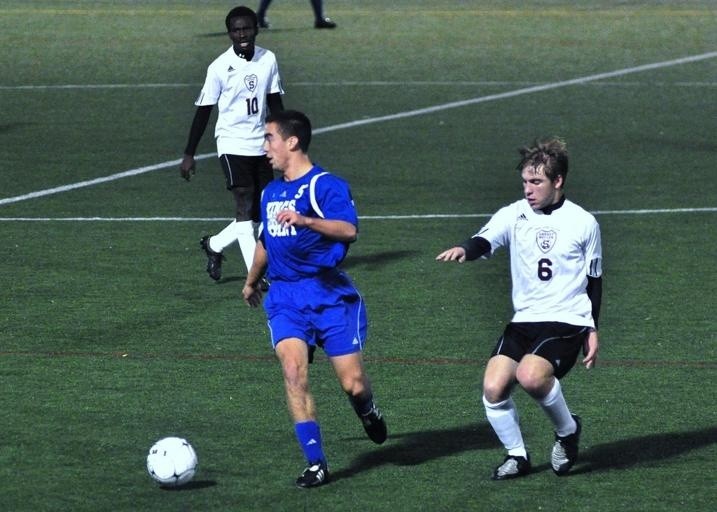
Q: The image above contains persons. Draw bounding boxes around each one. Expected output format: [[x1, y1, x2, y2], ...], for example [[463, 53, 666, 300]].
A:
[[241, 109, 389, 491], [433, 132, 606, 480], [254, 0, 338, 28], [179, 4, 286, 291]]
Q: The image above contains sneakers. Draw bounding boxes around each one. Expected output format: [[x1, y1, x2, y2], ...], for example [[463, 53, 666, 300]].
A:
[[200, 235, 223, 280], [296, 460, 329, 487], [551, 412, 582, 475], [491, 452, 530, 480], [315, 19, 336, 28], [256, 277, 270, 291], [255, 12, 269, 29], [351, 398, 386, 444]]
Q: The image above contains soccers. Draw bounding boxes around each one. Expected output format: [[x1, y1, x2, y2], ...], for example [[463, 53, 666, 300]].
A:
[[147, 436, 199, 489]]
